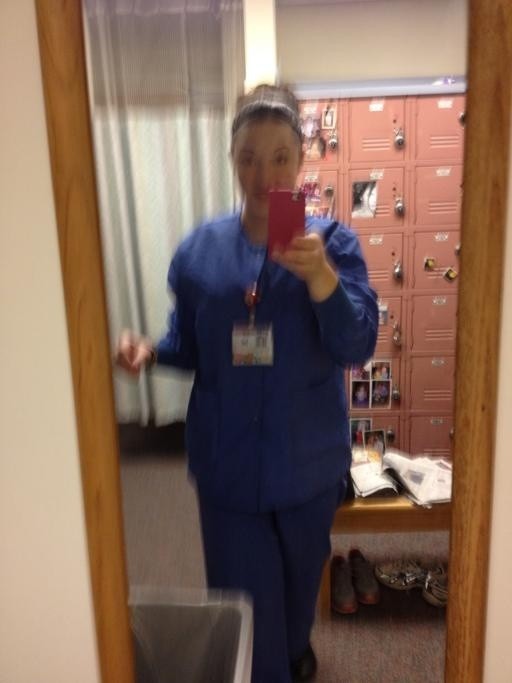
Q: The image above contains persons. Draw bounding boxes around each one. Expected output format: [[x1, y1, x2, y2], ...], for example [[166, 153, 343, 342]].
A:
[[117, 85, 378, 683]]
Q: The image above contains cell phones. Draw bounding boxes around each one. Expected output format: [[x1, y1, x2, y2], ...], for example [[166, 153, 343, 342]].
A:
[[268, 190, 304, 256]]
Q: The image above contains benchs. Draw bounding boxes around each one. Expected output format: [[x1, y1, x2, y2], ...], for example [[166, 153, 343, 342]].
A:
[[314, 486, 450, 619]]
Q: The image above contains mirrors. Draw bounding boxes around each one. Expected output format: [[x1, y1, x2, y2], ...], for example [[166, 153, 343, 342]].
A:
[[30, 1, 512, 683]]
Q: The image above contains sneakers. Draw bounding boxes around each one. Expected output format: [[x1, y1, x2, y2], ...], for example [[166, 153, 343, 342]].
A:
[[375, 557, 435, 590], [331, 556, 358, 614], [422, 551, 447, 608], [348, 550, 380, 605]]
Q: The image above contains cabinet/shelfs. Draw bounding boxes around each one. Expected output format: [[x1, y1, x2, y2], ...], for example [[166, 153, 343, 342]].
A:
[[297, 80, 466, 460]]
